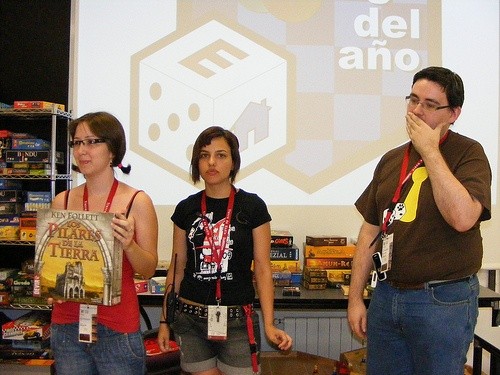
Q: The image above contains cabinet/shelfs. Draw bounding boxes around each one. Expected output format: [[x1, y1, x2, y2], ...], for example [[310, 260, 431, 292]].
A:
[[0, 108, 75, 316]]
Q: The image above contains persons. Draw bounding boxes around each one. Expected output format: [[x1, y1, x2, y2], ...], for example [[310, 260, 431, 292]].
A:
[[156, 125, 293, 375], [50, 111, 158, 375], [347, 67, 491, 375]]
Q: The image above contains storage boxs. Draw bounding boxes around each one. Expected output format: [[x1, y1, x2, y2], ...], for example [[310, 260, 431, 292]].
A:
[[0, 135, 64, 243], [0, 261, 51, 304], [249, 231, 360, 289], [4, 313, 55, 366]]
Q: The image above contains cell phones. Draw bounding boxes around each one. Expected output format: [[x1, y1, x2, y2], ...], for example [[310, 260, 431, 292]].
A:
[[371, 252, 387, 281]]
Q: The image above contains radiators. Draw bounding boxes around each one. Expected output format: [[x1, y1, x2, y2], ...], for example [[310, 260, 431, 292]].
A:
[[272, 312, 368, 360]]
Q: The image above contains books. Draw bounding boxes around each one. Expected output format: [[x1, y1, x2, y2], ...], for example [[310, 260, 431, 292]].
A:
[[303, 236, 358, 289], [250, 229, 302, 287]]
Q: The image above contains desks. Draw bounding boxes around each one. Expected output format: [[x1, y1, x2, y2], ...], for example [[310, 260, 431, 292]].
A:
[[139, 286, 499, 328]]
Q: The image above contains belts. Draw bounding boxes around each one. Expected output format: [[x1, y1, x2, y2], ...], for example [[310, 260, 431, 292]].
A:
[[176, 300, 254, 319], [389, 273, 473, 289]]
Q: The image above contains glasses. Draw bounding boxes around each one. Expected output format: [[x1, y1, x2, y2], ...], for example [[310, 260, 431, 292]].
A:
[[405, 96, 451, 112], [69, 139, 105, 148]]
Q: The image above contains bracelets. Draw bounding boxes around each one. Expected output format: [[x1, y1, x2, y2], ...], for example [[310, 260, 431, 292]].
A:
[[160, 321, 167, 323]]
